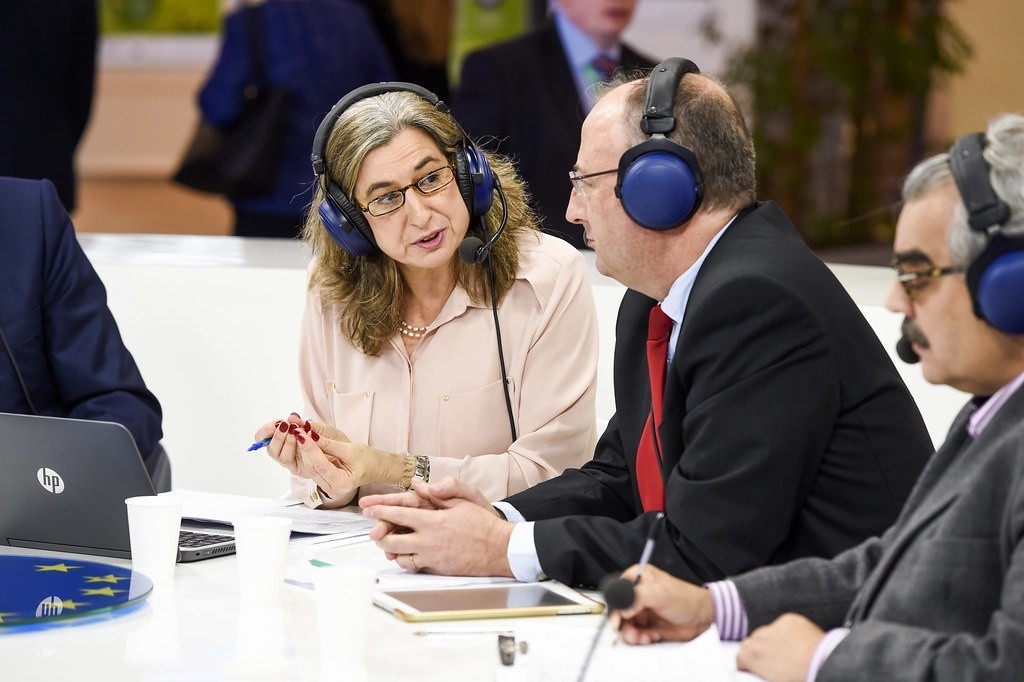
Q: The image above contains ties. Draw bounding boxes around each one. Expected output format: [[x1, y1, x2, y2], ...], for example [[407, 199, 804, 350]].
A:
[[635, 304, 672, 513], [595, 53, 617, 85]]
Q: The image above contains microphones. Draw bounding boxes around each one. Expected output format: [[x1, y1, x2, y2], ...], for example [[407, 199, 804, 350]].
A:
[[578, 578, 636, 682], [895, 336, 919, 364], [458, 185, 508, 264]]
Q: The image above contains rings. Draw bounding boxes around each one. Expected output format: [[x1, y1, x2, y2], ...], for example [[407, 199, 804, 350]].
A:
[[410, 554, 418, 571]]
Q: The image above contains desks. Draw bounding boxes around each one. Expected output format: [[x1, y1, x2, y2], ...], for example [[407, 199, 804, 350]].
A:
[[0, 509, 769, 682]]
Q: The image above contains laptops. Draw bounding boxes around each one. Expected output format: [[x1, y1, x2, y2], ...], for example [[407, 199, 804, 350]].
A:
[[0, 413, 236, 562]]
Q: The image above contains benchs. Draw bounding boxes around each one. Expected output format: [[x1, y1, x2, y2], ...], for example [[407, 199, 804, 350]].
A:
[[77, 234, 974, 501]]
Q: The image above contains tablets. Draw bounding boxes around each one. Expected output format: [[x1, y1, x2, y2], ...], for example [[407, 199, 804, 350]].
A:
[[371, 580, 606, 622]]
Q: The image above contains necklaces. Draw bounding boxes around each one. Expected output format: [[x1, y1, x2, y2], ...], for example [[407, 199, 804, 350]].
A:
[[399, 321, 428, 338]]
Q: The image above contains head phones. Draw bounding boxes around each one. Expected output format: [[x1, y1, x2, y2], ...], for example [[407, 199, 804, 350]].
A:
[[613, 57, 705, 231], [310, 81, 502, 258], [944, 131, 1024, 335]]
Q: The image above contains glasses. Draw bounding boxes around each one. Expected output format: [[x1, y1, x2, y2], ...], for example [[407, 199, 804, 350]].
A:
[[356, 165, 455, 217], [569, 167, 623, 197], [892, 255, 970, 295]]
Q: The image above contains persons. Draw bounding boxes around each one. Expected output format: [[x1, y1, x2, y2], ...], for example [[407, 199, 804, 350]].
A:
[[198, 0, 416, 236], [611, 115, 1024, 682], [0, 176, 163, 459], [1, 0, 103, 210], [254, 90, 599, 510], [358, 74, 935, 588], [461, 0, 660, 249]]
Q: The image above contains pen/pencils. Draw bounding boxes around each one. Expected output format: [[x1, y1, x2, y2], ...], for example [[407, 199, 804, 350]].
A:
[[611, 512, 665, 648], [247, 418, 314, 451]]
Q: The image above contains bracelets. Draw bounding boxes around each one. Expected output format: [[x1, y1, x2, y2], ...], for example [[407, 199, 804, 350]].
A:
[[391, 449, 430, 491]]
